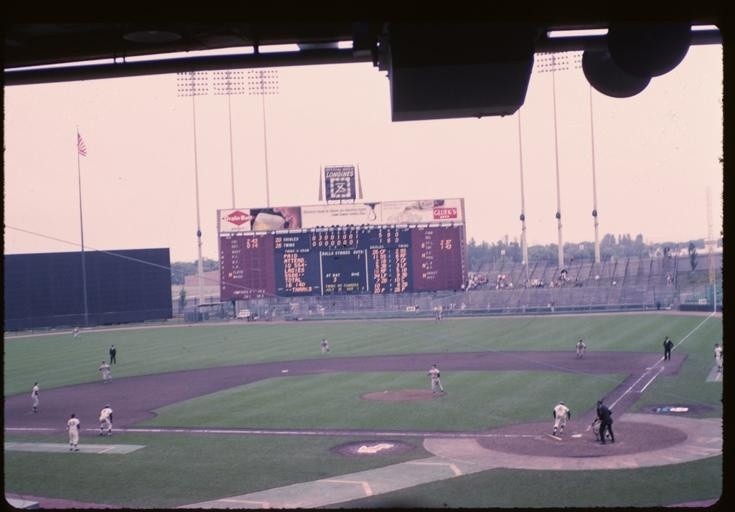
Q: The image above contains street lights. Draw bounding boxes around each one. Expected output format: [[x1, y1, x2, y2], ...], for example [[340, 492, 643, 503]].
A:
[[535, 51, 570, 270], [570, 49, 599, 266], [177, 69, 208, 318], [247, 66, 280, 207], [212, 69, 246, 209]]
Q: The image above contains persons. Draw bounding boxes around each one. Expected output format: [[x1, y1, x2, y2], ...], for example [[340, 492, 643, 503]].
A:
[[110, 344, 117, 364], [99, 403, 113, 436], [100, 361, 112, 384], [320, 338, 328, 353], [553, 401, 571, 433], [428, 364, 444, 393], [597, 399, 615, 443], [434, 273, 583, 320], [575, 339, 586, 358], [663, 336, 674, 360], [67, 413, 81, 451], [713, 343, 723, 370], [656, 269, 674, 311], [31, 381, 40, 412], [248, 297, 326, 320]]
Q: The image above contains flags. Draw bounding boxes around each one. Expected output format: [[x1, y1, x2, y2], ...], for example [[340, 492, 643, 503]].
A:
[[79, 134, 88, 157]]
